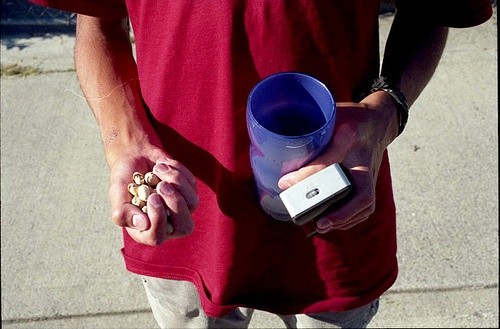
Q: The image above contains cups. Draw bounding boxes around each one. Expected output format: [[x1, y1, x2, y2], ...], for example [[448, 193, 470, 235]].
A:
[[245, 71, 336, 221]]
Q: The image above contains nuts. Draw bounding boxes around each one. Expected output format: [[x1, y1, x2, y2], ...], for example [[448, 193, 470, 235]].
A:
[[127, 172, 174, 234]]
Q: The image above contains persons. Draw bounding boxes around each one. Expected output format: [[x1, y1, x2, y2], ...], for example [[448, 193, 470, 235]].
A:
[[72, 1, 494, 329]]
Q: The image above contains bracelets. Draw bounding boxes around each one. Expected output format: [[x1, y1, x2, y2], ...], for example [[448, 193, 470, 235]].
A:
[[370, 76, 409, 137]]
